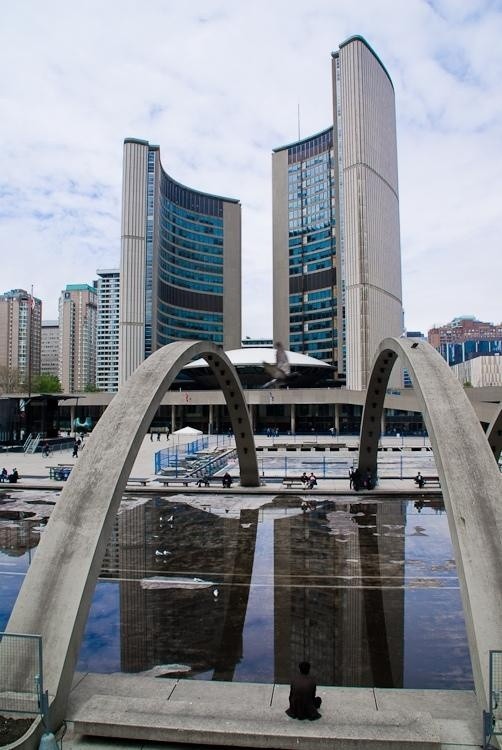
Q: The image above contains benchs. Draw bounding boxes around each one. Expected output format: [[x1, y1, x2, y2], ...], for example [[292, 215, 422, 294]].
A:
[[414, 500, 444, 508], [0, 476, 21, 483], [283, 477, 317, 489], [160, 479, 191, 487], [126, 478, 150, 486], [414, 477, 441, 488]]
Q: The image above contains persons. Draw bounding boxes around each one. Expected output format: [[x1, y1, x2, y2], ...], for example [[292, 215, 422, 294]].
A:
[[72, 437, 83, 459], [307, 471, 317, 488], [195, 472, 208, 488], [347, 465, 378, 491], [9, 467, 20, 483], [1, 466, 8, 482], [285, 662, 323, 722], [150, 429, 171, 442], [266, 424, 280, 438], [415, 471, 425, 489], [301, 473, 307, 489], [219, 472, 234, 488]]
